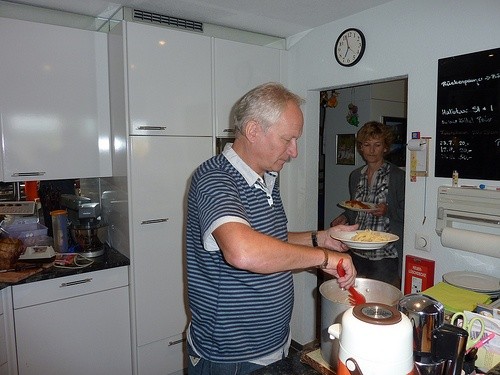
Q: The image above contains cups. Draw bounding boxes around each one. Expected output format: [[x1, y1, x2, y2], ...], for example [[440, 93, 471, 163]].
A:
[[51, 210, 69, 253]]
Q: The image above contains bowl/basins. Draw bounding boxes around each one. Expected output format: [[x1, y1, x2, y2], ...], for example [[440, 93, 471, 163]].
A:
[[69, 216, 107, 258]]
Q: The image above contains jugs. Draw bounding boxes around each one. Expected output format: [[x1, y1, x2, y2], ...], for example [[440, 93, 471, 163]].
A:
[[327, 302, 415, 375]]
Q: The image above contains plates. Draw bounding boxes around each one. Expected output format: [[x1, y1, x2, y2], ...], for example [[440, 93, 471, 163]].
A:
[[442, 271, 500, 294], [338, 201, 380, 213], [330, 229, 400, 250]]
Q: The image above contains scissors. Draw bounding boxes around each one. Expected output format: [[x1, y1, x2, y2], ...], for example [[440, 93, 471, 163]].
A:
[[450, 312, 484, 351]]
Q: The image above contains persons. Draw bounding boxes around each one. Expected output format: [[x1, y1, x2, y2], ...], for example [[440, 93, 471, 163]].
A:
[[330, 120, 406, 284], [186, 82, 357, 375]]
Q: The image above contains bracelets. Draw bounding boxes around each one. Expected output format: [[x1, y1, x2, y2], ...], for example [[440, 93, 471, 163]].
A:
[[312, 231, 318, 247], [314, 248, 328, 269]]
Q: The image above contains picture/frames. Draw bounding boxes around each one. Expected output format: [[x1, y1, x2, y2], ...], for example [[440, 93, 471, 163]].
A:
[[335, 133, 356, 166]]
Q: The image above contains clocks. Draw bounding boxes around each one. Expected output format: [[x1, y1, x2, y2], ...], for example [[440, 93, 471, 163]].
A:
[[334, 28, 367, 67]]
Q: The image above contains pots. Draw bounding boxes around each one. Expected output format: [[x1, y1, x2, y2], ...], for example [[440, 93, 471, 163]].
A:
[[319, 277, 404, 368]]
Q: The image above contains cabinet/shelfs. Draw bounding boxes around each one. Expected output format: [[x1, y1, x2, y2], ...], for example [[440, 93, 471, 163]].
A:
[[0, 15, 281, 375]]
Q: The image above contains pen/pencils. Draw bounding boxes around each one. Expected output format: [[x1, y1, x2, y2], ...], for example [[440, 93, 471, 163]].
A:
[[465, 333, 495, 354], [464, 347, 478, 365]]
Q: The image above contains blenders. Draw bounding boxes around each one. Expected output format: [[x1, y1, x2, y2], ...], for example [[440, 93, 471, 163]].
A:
[[395, 292, 468, 375]]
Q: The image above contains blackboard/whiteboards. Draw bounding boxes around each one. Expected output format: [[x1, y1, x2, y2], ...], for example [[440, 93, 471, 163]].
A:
[[434, 47, 500, 181]]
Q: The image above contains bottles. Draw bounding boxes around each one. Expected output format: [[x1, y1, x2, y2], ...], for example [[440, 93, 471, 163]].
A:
[[452, 170, 458, 188]]
